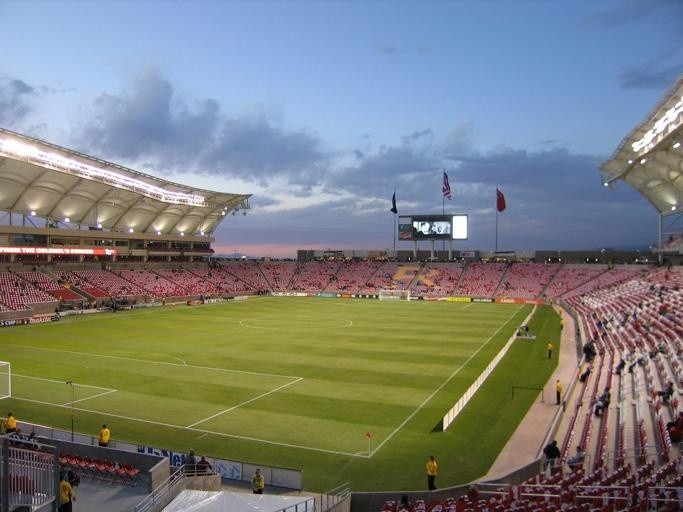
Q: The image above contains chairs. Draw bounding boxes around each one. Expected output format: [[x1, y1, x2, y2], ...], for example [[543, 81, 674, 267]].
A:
[[2, 433, 143, 486], [376, 458, 682, 511], [538, 260, 682, 466], [1, 261, 274, 315], [257, 261, 562, 303]]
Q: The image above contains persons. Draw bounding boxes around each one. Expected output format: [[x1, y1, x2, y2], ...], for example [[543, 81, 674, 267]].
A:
[[426, 456, 438, 490], [397, 495, 411, 510], [59, 472, 77, 511], [182, 451, 196, 477], [252, 468, 264, 495], [14, 263, 184, 315], [184, 262, 467, 300], [4, 412, 38, 450], [98, 424, 110, 447], [466, 261, 683, 503], [196, 456, 214, 477]]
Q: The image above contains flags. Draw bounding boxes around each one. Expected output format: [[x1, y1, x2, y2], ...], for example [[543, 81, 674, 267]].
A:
[[496, 188, 505, 212], [442, 173, 451, 200], [390, 192, 397, 213]]
